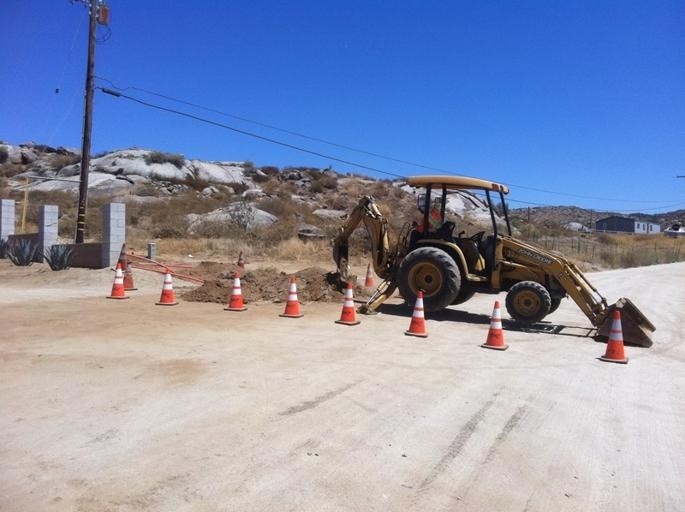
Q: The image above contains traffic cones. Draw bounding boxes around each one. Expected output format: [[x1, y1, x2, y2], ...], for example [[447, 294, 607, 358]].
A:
[[279, 274, 304, 319], [597, 310, 629, 365], [238, 252, 247, 267], [156, 268, 179, 306], [404, 287, 431, 337], [364, 260, 376, 289], [335, 281, 360, 326], [107, 261, 134, 299], [223, 272, 247, 312], [481, 300, 508, 350]]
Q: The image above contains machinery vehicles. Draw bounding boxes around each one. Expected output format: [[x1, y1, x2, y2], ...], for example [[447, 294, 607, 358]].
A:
[[332, 176, 656, 348]]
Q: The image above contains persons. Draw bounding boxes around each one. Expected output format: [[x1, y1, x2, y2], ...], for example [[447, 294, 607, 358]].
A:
[[408, 207, 433, 253]]
